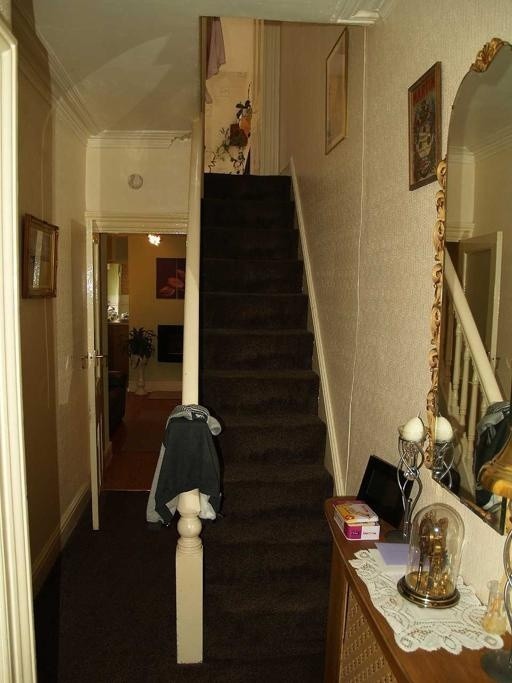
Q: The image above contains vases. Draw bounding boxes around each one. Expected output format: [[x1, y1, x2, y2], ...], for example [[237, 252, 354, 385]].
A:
[[134, 353, 148, 396]]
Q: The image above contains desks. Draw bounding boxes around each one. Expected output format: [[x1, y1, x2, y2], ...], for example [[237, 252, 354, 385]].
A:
[[324, 494, 512, 683]]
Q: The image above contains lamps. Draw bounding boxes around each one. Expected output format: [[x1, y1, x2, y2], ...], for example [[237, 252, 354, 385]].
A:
[[384, 416, 425, 543], [434, 414, 454, 489]]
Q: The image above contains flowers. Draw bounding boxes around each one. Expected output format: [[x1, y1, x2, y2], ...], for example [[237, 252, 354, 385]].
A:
[[122, 328, 155, 358]]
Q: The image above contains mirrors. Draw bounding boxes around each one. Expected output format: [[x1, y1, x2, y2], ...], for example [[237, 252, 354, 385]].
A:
[[420, 38, 512, 531]]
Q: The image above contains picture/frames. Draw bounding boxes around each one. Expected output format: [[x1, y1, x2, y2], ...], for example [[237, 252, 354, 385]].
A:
[[325, 25, 347, 155], [23, 212, 58, 299], [408, 61, 442, 190]]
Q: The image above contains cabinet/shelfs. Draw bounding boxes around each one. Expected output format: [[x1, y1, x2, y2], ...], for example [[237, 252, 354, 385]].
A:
[[107, 323, 130, 389]]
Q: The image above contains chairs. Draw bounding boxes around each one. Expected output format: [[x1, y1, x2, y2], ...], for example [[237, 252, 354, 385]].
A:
[[107, 369, 128, 434]]
